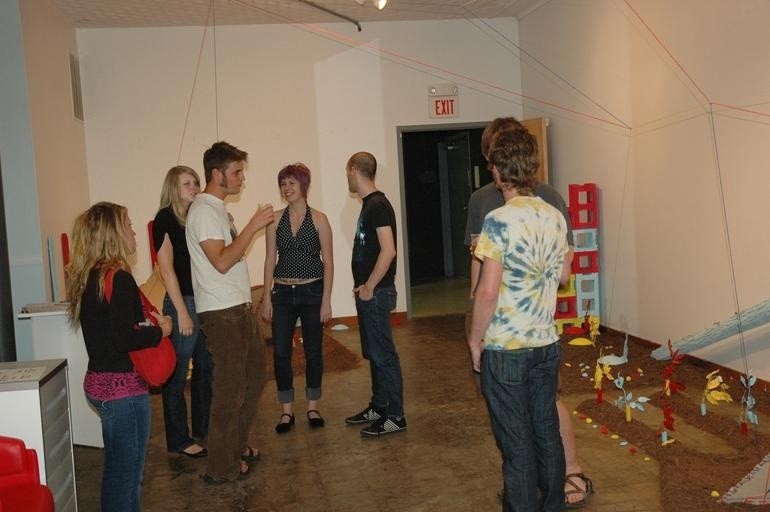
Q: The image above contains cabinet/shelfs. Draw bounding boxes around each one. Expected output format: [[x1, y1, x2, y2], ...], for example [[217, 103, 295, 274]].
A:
[[0, 359, 78, 511], [16, 304, 106, 450]]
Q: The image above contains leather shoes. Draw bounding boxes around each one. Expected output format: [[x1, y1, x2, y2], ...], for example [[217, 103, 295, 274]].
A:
[[177, 440, 209, 458]]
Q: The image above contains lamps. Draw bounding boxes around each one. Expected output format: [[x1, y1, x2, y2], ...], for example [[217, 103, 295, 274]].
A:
[[355, 0, 388, 11]]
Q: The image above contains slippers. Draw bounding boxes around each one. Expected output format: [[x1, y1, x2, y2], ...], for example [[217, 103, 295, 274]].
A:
[[242, 446, 263, 462], [202, 459, 251, 485]]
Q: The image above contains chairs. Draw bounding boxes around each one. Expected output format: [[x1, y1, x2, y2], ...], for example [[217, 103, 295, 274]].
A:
[[0, 436, 54, 511]]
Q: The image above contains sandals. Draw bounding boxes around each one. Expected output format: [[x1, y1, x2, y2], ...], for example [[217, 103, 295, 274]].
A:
[[566, 470, 595, 508]]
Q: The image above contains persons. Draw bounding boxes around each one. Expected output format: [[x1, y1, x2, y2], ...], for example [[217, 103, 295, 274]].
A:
[[64, 200, 172, 512], [258, 163, 333, 435], [151, 166, 213, 461], [185, 141, 275, 483], [345, 151, 409, 440]]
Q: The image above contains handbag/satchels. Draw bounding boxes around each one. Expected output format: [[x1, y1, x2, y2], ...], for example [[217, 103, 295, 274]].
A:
[[104, 267, 179, 388]]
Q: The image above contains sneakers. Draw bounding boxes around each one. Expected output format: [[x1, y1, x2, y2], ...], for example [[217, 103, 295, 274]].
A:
[[345, 404, 389, 424], [306, 410, 325, 426], [275, 411, 296, 434], [360, 417, 408, 438]]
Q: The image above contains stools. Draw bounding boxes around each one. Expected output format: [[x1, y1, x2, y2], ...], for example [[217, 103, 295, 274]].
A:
[[567, 182, 599, 229], [572, 252, 600, 274], [555, 318, 585, 336], [575, 274, 600, 317], [554, 298, 577, 320], [557, 275, 577, 298], [571, 230, 599, 253]]
